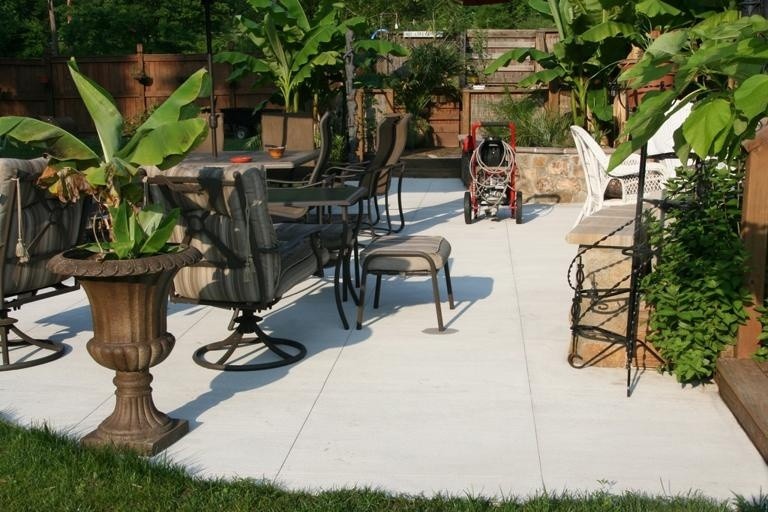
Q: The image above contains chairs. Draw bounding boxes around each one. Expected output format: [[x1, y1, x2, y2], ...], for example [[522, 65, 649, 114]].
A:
[[1, 111, 411, 329], [646, 99, 737, 192], [567, 124, 670, 232], [138, 161, 330, 371], [2, 157, 92, 370]]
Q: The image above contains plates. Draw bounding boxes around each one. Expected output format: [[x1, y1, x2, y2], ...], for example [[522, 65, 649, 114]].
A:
[[229, 156, 252, 163]]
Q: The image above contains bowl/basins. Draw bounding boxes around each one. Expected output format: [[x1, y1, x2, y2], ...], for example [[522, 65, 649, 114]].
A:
[[268, 146, 285, 159]]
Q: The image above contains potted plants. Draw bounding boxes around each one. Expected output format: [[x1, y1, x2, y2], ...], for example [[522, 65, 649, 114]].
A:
[[0, 57, 213, 458]]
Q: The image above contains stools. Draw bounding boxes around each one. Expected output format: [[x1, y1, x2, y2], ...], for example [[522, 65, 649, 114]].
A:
[[357, 235, 455, 331]]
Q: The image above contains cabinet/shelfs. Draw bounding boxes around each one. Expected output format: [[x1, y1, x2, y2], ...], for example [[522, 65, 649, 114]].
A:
[[565, 203, 676, 368]]
